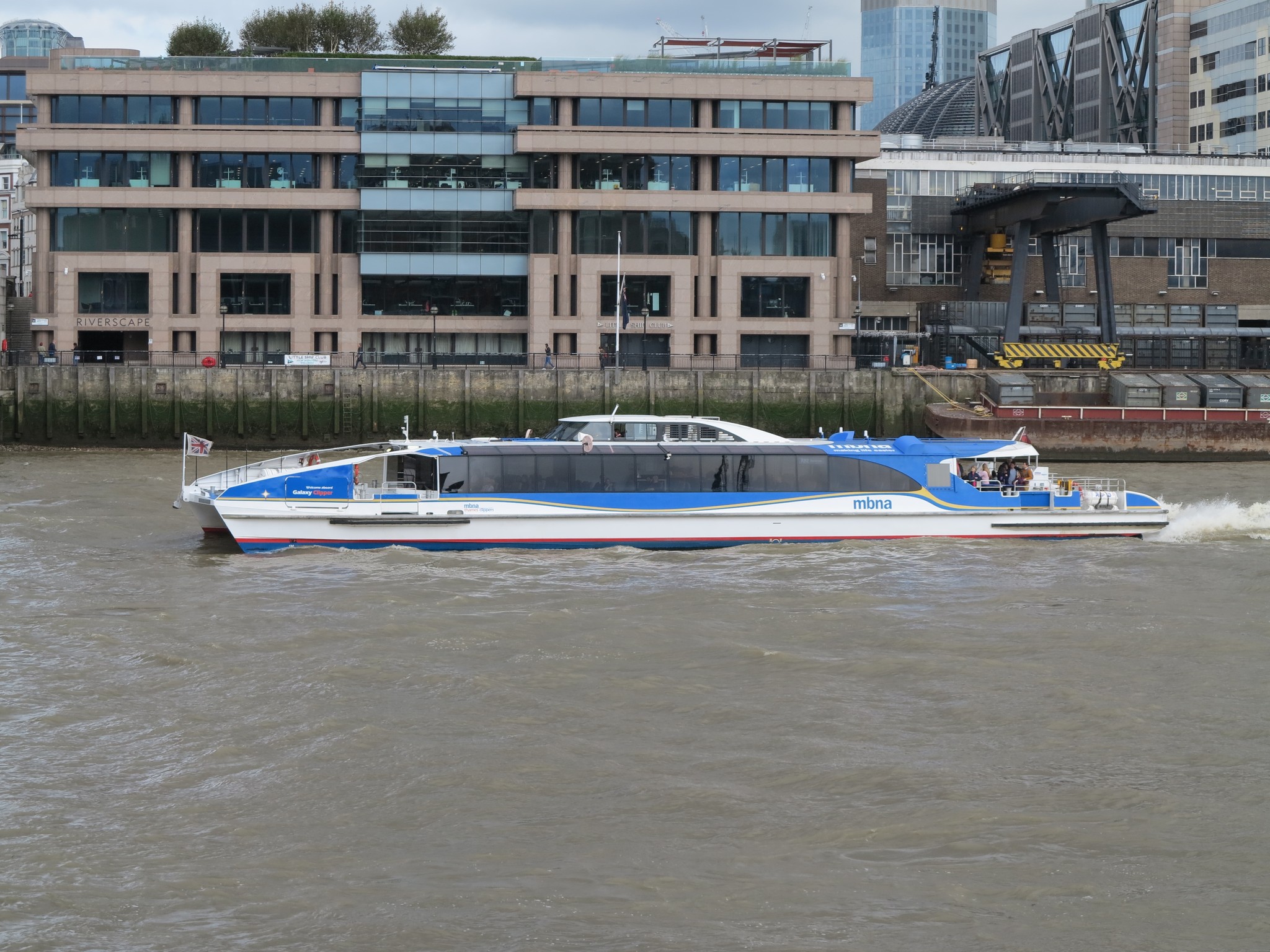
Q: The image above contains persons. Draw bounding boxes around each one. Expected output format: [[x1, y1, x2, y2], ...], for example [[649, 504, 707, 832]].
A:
[[598, 346, 605, 370], [478, 458, 1035, 490], [70, 343, 80, 366], [47, 339, 56, 367], [37, 342, 46, 367], [541, 343, 555, 370], [353, 343, 367, 369]]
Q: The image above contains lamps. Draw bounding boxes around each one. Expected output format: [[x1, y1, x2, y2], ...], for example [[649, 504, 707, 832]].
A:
[[851, 275, 857, 282], [1035, 290, 1044, 293], [1159, 291, 1167, 295], [25, 268, 32, 276], [1211, 291, 1220, 295], [821, 273, 826, 280], [890, 288, 898, 291], [64, 268, 68, 275], [1089, 290, 1098, 294]]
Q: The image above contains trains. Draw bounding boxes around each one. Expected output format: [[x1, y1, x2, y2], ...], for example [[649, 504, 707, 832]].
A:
[[922, 326, 1270, 372]]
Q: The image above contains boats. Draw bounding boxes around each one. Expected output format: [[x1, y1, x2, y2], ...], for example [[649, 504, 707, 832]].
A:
[[924, 370, 1270, 464], [170, 402, 1169, 554]]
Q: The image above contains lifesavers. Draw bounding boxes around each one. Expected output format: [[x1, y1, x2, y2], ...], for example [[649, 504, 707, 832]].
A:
[[1072, 482, 1082, 498], [308, 455, 320, 467], [354, 464, 360, 485]]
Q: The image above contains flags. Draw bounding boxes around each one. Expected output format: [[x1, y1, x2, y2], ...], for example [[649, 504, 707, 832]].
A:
[[619, 278, 629, 329], [186, 434, 214, 457]]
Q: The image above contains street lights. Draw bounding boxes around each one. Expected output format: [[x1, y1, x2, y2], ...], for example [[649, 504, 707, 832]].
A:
[[7, 301, 14, 366], [875, 316, 882, 332], [854, 306, 862, 371], [430, 304, 438, 369], [640, 305, 650, 371], [220, 302, 228, 369]]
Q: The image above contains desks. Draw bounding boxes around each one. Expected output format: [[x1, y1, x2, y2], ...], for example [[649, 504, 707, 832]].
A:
[[249, 304, 265, 314], [450, 305, 475, 316], [766, 307, 791, 318], [273, 304, 283, 315], [502, 305, 525, 316], [221, 304, 242, 315], [614, 305, 639, 316], [362, 304, 375, 315], [398, 305, 423, 315]]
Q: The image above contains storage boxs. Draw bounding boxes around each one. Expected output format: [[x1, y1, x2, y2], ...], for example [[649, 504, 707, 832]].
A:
[[919, 301, 1270, 409]]
